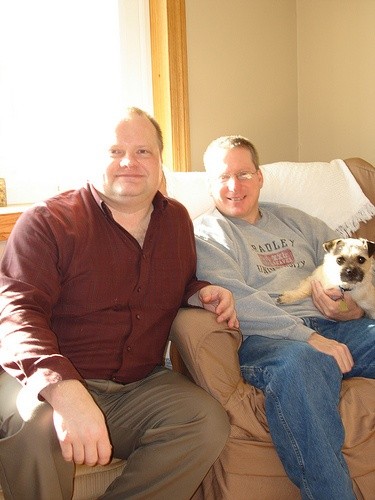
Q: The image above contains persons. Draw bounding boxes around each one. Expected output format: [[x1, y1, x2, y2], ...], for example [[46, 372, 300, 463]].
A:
[[0, 108, 238, 500], [192, 136, 375, 500]]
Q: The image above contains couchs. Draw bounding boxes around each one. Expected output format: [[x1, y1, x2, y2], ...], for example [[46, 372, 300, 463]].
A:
[[165, 155, 375, 500]]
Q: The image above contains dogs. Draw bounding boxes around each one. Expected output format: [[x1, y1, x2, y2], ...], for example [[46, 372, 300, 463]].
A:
[[276, 237, 374, 322]]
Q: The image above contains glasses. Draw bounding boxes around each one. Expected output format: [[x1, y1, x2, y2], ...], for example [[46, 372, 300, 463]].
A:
[[214, 170, 259, 183]]
